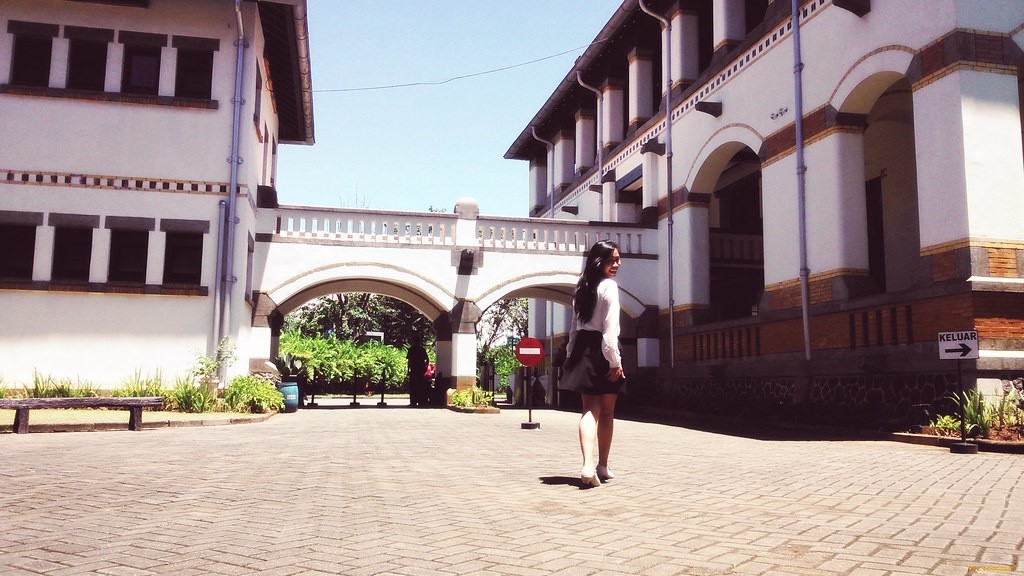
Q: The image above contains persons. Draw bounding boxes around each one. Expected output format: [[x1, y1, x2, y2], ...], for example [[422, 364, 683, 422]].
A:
[[407, 336, 429, 407], [564, 239, 625, 486], [424, 360, 435, 387], [476, 376, 480, 387]]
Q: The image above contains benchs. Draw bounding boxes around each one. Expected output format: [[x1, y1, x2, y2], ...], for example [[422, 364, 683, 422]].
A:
[[0, 397, 163, 435]]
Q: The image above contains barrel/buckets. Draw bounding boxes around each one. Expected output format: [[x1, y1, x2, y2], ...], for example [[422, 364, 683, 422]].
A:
[[277, 382, 298, 413]]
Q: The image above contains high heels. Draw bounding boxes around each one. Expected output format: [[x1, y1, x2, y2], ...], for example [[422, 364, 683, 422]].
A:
[[596, 465, 614, 482], [581, 466, 601, 487]]
